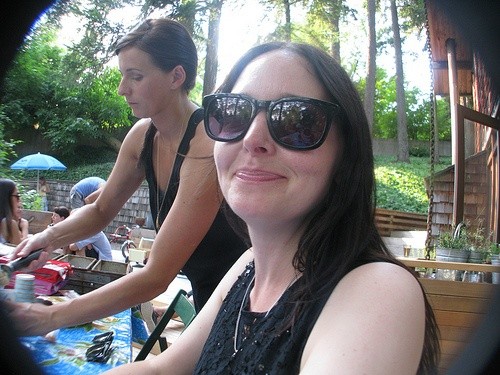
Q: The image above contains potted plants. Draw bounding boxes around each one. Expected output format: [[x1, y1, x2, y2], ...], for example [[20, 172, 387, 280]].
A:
[[432, 216, 500, 285]]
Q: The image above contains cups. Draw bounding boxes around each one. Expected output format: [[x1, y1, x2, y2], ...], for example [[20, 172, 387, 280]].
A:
[[404, 244, 411, 257], [14, 274, 35, 304]]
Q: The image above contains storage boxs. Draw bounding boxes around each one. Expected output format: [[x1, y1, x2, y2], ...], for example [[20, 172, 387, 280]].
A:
[[46, 238, 156, 276]]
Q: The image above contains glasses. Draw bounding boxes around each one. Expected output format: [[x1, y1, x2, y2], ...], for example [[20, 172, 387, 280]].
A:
[[202, 93, 343, 151], [86, 331, 120, 365], [12, 195, 20, 200]]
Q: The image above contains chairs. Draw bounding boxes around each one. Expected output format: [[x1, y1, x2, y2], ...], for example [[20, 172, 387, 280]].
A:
[[135, 289, 196, 362]]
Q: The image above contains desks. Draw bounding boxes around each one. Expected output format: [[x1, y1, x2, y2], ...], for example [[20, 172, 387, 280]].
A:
[[1, 288, 131, 375]]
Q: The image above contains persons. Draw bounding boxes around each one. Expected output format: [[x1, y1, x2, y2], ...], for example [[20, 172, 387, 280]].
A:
[[0, 293, 48, 375], [98, 42, 443, 375], [69, 209, 113, 260], [39, 176, 48, 210], [47, 205, 75, 255], [70, 177, 106, 209], [0, 178, 28, 245], [0, 19, 252, 338]]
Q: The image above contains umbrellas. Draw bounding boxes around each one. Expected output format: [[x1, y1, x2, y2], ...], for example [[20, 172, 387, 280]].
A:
[[11, 152, 67, 192]]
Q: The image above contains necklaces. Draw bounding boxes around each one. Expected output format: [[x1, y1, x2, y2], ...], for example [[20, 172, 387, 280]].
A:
[[156, 106, 191, 232]]
